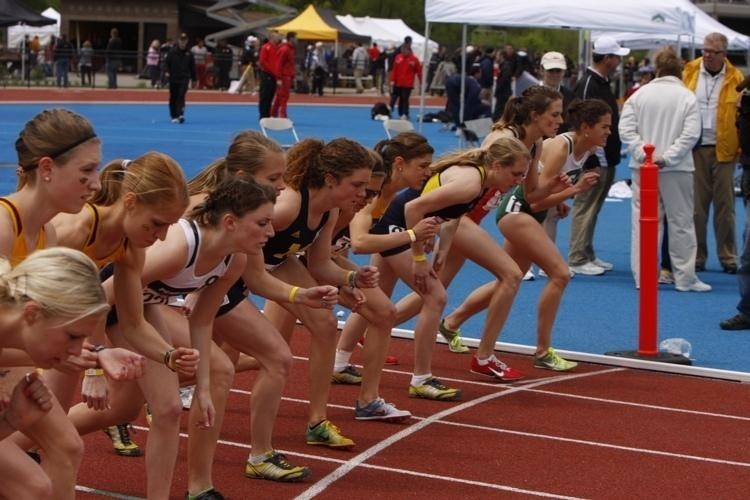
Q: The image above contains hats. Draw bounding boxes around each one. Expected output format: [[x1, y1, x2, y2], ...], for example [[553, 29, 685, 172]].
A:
[[592, 36, 630, 57], [541, 51, 567, 70]]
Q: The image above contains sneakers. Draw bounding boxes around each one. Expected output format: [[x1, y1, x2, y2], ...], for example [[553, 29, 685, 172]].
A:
[[469, 355, 526, 381], [524, 258, 748, 331], [172, 119, 179, 123], [408, 377, 461, 400], [246, 449, 309, 481], [533, 347, 578, 370], [439, 318, 470, 352], [177, 386, 194, 408], [354, 398, 412, 421], [180, 116, 185, 122], [306, 420, 354, 447], [184, 489, 224, 500], [329, 365, 361, 383], [355, 328, 398, 365], [102, 423, 141, 456], [143, 401, 152, 425]]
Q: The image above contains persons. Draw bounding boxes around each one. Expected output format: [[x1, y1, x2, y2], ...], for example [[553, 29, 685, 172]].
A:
[[1, 84, 614, 500], [0, 0, 750, 344]]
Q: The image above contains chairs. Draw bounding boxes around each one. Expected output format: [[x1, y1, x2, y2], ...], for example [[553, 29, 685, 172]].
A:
[[259, 117, 300, 151], [463, 117, 495, 149], [384, 119, 416, 141]]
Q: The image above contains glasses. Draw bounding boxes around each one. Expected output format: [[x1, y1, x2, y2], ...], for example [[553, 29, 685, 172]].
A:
[[701, 48, 725, 54]]
